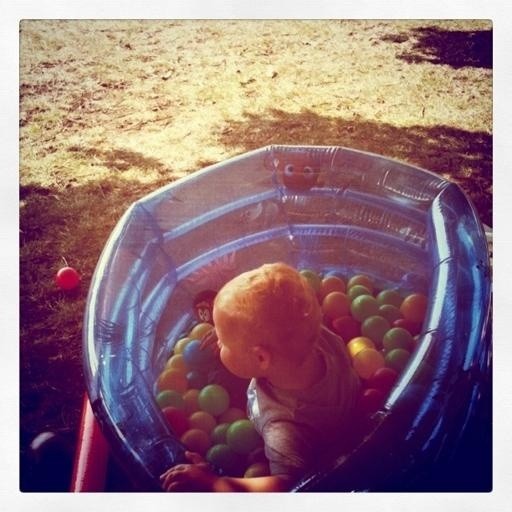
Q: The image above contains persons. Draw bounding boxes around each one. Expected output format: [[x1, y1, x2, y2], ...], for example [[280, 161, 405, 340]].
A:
[[158, 261, 362, 492]]
